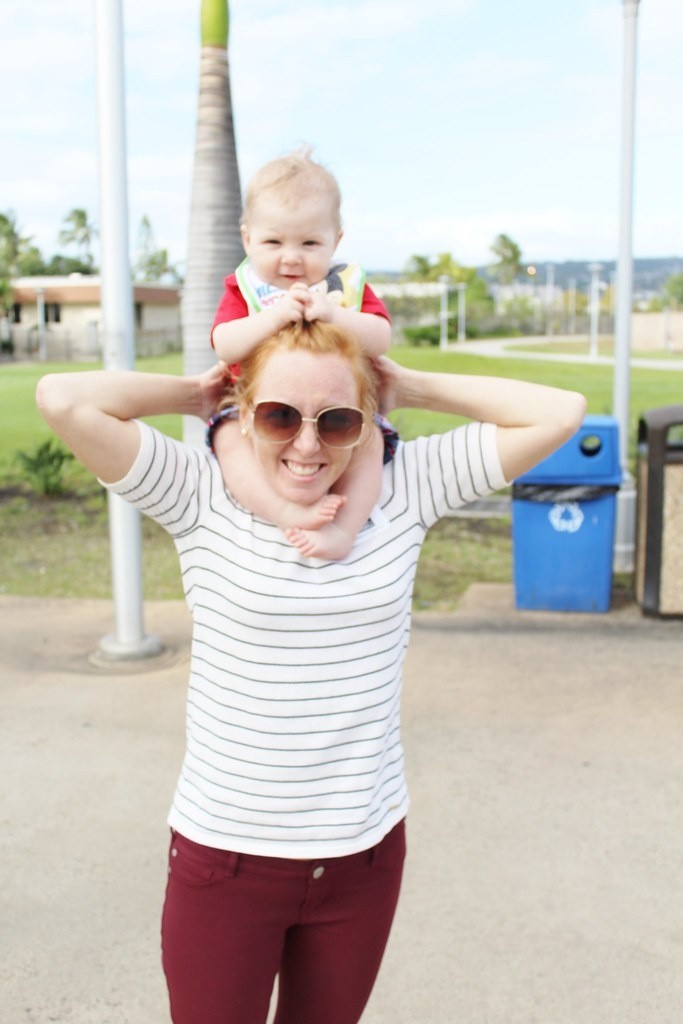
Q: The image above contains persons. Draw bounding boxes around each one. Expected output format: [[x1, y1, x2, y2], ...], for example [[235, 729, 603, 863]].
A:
[[202, 143, 398, 560], [37, 321, 586, 1024]]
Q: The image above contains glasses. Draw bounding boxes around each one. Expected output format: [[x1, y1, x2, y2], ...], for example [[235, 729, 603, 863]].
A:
[[245, 401, 369, 451]]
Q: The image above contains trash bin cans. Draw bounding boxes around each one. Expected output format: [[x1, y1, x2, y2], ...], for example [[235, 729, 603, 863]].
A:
[[510, 416, 625, 614], [631, 404, 683, 619]]
